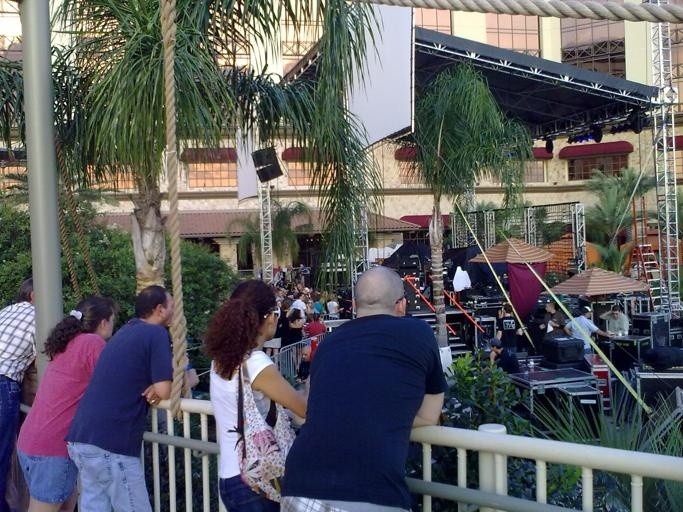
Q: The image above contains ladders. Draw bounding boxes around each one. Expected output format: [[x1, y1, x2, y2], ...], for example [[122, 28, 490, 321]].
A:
[[624, 196, 667, 311]]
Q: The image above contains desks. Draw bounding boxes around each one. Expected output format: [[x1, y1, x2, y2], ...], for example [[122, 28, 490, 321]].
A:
[[506, 367, 599, 414], [635, 367, 683, 429]]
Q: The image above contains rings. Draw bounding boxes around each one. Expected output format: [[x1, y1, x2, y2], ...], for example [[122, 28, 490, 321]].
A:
[[152, 399, 156, 404]]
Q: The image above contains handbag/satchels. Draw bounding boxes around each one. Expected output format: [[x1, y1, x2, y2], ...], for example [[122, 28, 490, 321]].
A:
[[239, 360, 298, 503]]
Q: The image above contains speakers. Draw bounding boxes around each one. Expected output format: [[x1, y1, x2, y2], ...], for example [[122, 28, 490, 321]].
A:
[[250, 147, 283, 184]]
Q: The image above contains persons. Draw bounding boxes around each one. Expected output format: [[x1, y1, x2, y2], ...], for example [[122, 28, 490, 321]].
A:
[[277, 264, 354, 384], [203, 279, 308, 512], [539, 302, 566, 338], [564, 306, 614, 354], [280, 265, 449, 512], [63, 285, 174, 512], [498, 305, 523, 352], [599, 305, 629, 371], [0, 278, 37, 512], [489, 338, 520, 374], [392, 268, 450, 310], [16, 297, 115, 511]]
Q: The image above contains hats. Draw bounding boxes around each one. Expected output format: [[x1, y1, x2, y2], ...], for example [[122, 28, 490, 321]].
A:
[[581, 306, 593, 312], [489, 338, 502, 345]]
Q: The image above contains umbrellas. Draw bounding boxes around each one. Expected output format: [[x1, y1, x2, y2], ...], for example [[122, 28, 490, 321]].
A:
[[539, 267, 651, 298], [469, 237, 555, 263]]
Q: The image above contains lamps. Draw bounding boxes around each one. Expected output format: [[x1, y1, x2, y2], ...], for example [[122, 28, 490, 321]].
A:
[[566, 113, 651, 144]]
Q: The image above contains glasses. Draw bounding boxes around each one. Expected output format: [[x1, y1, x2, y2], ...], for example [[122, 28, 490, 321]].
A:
[[262, 306, 281, 319]]
